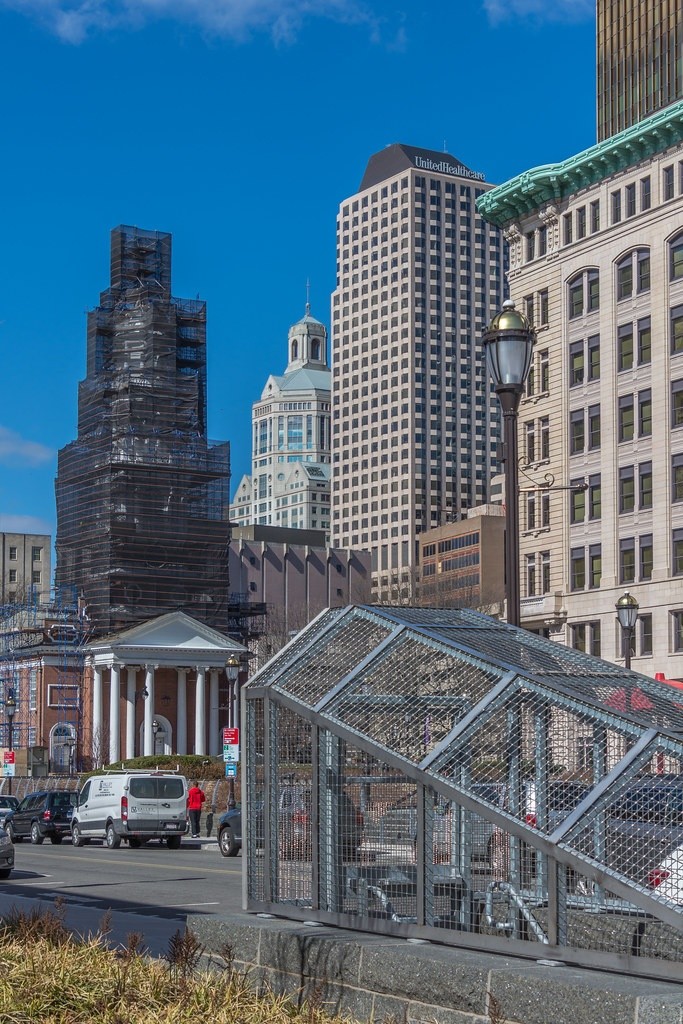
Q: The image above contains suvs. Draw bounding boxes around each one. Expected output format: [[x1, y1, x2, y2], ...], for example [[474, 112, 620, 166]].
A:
[[551, 774, 683, 907], [3, 788, 78, 845]]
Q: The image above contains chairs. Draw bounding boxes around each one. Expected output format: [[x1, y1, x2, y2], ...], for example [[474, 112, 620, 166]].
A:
[[59, 800, 67, 805]]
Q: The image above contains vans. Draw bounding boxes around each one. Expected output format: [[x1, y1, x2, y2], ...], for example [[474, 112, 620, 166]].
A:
[[355, 777, 592, 899], [69, 762, 190, 848]]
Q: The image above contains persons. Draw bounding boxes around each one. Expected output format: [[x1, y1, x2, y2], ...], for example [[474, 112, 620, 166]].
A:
[[187, 781, 206, 838]]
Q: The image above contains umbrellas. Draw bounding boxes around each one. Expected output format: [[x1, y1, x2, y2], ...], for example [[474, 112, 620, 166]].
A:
[[603, 673, 683, 774]]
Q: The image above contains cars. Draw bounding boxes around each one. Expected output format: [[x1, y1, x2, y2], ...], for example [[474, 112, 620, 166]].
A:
[[216, 789, 364, 857], [0, 827, 15, 877], [0, 795, 19, 836]]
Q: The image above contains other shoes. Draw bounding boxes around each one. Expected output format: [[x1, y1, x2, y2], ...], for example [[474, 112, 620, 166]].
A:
[[196, 835, 199, 837], [191, 835, 196, 838]]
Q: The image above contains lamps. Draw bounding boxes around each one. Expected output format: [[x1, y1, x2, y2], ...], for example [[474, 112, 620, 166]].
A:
[[161, 669, 171, 707], [141, 686, 149, 700]]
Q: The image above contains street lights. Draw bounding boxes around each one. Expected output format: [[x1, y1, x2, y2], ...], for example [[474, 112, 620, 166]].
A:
[[4, 696, 16, 794], [225, 653, 241, 811], [478, 297, 538, 941], [613, 590, 640, 758]]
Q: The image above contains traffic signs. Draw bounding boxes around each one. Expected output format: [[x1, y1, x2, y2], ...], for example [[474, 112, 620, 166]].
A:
[[222, 727, 240, 744], [3, 751, 15, 764]]
